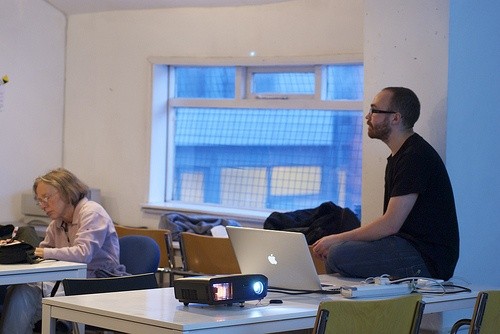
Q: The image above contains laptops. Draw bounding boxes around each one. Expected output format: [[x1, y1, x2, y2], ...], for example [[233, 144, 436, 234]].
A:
[[224, 225, 357, 294]]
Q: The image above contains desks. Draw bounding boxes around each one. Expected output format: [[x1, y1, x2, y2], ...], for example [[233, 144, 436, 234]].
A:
[[0, 259, 87, 334], [5, 222, 181, 251], [41, 271, 479, 334]]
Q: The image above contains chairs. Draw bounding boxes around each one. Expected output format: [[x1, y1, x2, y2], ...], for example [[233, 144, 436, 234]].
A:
[[311, 294, 425, 333], [119, 235, 161, 275], [63, 271, 159, 334], [449, 290, 500, 334], [180, 231, 241, 274], [112, 224, 176, 285]]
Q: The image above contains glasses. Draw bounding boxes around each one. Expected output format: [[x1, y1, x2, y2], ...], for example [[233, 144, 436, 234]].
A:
[[369, 107, 396, 116], [35, 191, 58, 208]]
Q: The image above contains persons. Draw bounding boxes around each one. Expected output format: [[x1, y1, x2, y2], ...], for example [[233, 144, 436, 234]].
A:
[[0, 167, 119, 334], [312, 87, 460, 281]]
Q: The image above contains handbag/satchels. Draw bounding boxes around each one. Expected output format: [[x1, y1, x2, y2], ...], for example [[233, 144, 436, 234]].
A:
[[0, 243, 37, 264]]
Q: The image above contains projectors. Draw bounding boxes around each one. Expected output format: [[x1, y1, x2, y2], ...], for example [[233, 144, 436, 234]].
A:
[[173, 272, 269, 306]]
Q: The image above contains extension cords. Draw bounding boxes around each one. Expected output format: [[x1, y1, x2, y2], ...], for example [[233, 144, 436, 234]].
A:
[[342, 283, 415, 298]]
